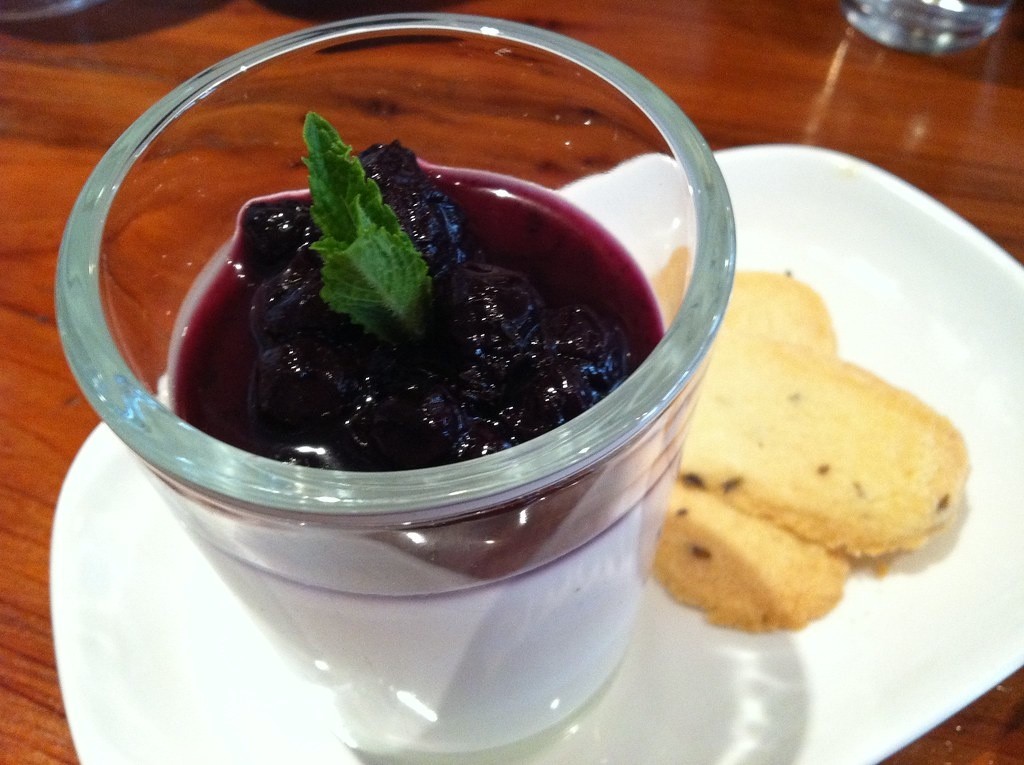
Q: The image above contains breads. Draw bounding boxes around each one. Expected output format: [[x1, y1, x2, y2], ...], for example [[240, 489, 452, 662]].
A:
[[648, 246, 970, 632]]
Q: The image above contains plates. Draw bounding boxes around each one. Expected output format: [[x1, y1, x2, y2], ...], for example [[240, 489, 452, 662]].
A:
[[49, 144, 1024, 765]]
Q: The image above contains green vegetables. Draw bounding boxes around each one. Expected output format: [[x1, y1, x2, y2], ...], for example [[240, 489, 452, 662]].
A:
[[301, 111, 434, 344]]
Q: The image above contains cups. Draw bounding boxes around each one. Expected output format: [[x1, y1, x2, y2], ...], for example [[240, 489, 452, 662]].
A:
[[55, 12, 736, 758], [838, 1, 1010, 56]]
